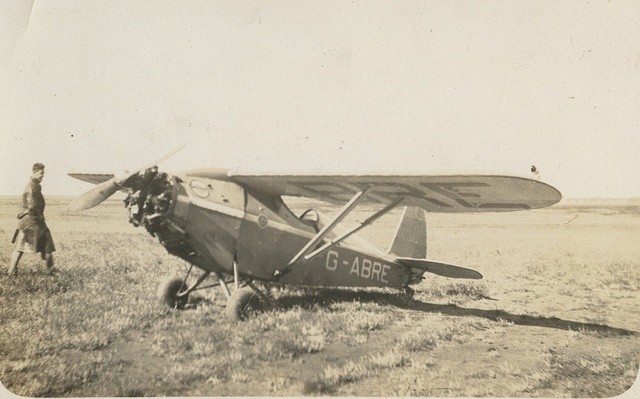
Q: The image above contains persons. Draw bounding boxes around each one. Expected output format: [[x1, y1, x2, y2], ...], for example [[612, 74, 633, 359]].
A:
[[7, 162, 56, 275]]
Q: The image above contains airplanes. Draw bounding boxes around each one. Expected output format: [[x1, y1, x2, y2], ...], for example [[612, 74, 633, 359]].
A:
[[68, 164, 562, 320]]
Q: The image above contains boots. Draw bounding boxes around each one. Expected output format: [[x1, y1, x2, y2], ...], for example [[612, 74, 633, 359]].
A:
[[39, 253, 56, 274], [7, 251, 24, 274]]
